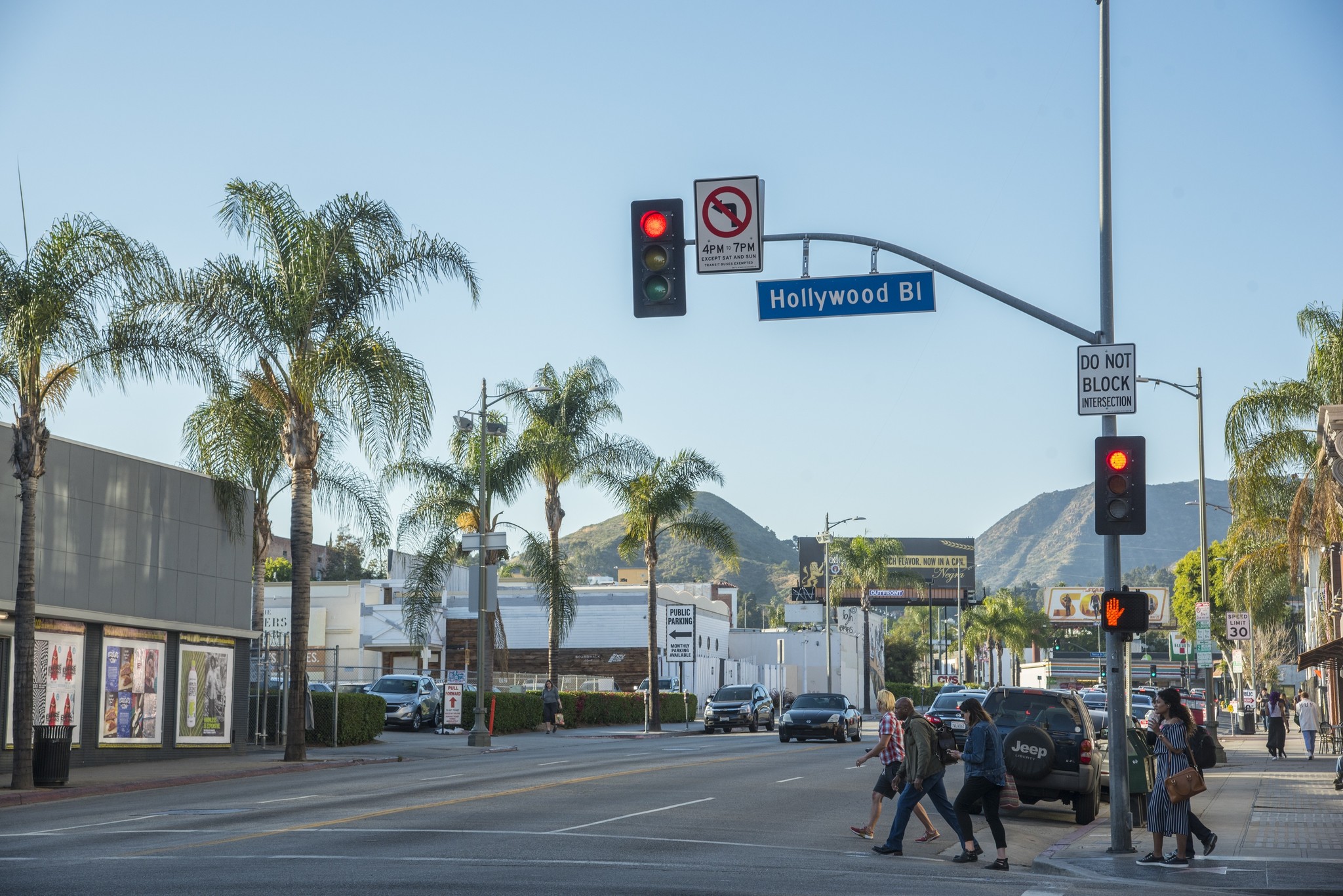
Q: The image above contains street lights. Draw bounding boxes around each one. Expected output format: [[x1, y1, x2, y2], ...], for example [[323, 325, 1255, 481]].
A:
[[925, 578, 935, 687], [1137, 368, 1227, 763], [1186, 500, 1260, 733], [1012, 587, 1038, 686], [825, 512, 867, 693], [958, 562, 987, 685], [468, 379, 550, 747]]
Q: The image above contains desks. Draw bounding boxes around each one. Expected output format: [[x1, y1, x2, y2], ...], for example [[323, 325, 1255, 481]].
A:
[[1319, 725, 1343, 754]]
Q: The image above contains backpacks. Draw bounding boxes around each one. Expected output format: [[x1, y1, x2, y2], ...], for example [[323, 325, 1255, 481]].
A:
[[910, 715, 959, 766]]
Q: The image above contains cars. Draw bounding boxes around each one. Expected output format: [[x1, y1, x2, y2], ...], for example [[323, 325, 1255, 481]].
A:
[[779, 693, 862, 743], [924, 683, 1219, 788], [256, 672, 501, 720]]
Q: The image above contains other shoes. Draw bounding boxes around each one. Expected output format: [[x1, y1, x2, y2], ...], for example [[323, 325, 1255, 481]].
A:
[[1298, 730, 1301, 733], [1272, 757, 1277, 760], [1312, 755, 1314, 759], [1308, 752, 1312, 760], [553, 725, 557, 733], [1279, 756, 1284, 759], [546, 730, 550, 734]]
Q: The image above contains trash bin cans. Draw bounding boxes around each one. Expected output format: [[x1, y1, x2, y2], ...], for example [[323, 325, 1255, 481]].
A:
[[33, 723, 77, 786], [1238, 707, 1256, 734]]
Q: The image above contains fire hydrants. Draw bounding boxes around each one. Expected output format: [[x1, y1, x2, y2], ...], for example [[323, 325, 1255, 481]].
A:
[[1220, 701, 1225, 710]]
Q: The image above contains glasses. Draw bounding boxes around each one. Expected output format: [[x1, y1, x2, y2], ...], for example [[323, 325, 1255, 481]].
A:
[[960, 710, 968, 717]]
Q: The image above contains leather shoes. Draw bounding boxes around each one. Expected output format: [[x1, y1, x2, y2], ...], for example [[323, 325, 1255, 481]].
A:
[[984, 858, 1009, 871], [872, 844, 903, 856], [953, 849, 978, 863], [954, 844, 984, 858]]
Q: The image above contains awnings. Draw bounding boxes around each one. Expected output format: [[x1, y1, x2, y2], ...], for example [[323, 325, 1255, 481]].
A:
[[1297, 637, 1343, 672]]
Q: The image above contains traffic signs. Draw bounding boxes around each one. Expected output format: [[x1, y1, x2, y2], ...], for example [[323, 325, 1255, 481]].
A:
[[667, 604, 695, 663], [1235, 689, 1258, 725], [445, 685, 462, 725]]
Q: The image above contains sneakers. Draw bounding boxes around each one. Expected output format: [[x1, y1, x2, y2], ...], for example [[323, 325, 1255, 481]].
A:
[[1165, 851, 1190, 859], [849, 823, 874, 839], [1201, 833, 1217, 856], [915, 829, 940, 843], [1159, 855, 1189, 868], [1136, 852, 1165, 866]]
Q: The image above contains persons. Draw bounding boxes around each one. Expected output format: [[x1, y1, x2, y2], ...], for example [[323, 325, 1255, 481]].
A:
[[1256, 688, 1270, 732], [849, 690, 940, 843], [946, 698, 1009, 871], [539, 680, 562, 734], [1062, 594, 1071, 616], [1296, 691, 1320, 760], [1293, 689, 1302, 733], [144, 650, 157, 693], [1333, 755, 1343, 784], [829, 698, 835, 706], [1279, 693, 1291, 732], [872, 697, 983, 856], [1136, 688, 1217, 868], [1090, 591, 1101, 619], [1149, 596, 1156, 614], [1265, 691, 1290, 760], [204, 655, 221, 717]]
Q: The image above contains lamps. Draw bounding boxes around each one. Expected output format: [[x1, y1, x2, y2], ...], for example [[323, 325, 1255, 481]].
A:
[[1327, 587, 1343, 617]]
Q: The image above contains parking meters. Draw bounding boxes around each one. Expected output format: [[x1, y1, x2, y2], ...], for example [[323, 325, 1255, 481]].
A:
[[684, 689, 689, 731], [644, 689, 650, 733]]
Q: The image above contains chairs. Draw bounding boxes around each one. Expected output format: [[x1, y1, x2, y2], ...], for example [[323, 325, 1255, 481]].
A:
[[806, 698, 819, 708], [945, 688, 952, 693], [1318, 721, 1343, 755], [948, 699, 958, 709], [725, 691, 735, 701], [1052, 714, 1075, 733], [995, 713, 1020, 727]]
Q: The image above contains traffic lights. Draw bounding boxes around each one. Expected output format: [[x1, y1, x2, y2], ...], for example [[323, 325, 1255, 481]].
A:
[[1100, 591, 1149, 635], [1095, 437, 1146, 535], [1150, 665, 1156, 678], [1100, 665, 1106, 677], [1152, 682, 1157, 686], [630, 198, 687, 318], [1054, 638, 1060, 651]]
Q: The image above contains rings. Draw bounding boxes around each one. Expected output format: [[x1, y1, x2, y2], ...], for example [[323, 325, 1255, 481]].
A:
[[857, 762, 859, 763]]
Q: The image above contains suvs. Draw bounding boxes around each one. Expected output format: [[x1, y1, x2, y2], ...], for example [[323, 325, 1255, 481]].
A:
[[633, 676, 684, 693], [704, 683, 775, 734], [578, 679, 622, 693], [364, 674, 441, 731], [521, 678, 547, 690], [980, 683, 1102, 824]]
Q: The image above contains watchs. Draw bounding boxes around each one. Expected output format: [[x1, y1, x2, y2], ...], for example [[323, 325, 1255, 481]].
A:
[[1159, 734, 1165, 740]]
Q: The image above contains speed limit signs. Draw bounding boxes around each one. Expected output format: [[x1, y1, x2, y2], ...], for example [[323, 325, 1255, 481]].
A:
[[1225, 612, 1251, 640]]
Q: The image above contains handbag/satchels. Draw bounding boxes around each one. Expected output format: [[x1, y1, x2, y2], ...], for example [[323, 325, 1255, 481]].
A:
[[1256, 708, 1263, 716], [1294, 714, 1300, 726], [554, 709, 565, 725], [1188, 723, 1217, 769], [999, 769, 1020, 810], [1164, 767, 1207, 804]]
